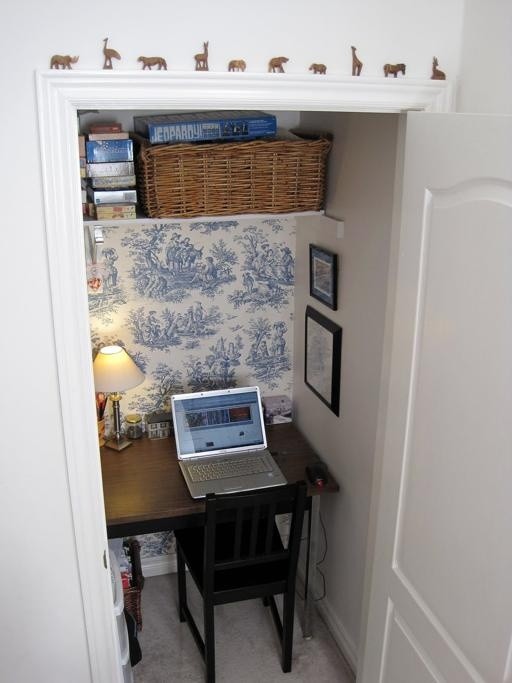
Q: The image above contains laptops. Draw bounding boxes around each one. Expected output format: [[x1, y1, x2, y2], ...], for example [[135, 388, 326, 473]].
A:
[[171, 385, 288, 499]]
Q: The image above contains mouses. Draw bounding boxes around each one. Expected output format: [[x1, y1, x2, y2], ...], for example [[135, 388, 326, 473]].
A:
[[306, 460, 328, 488]]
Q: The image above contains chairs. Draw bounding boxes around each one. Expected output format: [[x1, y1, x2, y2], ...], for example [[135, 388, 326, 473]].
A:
[[173, 479, 308, 682]]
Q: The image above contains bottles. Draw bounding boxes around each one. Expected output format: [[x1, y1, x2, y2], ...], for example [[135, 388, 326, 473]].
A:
[[125, 413, 143, 438]]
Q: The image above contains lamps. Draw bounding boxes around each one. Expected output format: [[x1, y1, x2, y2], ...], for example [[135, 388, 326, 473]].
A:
[[93, 346, 145, 451]]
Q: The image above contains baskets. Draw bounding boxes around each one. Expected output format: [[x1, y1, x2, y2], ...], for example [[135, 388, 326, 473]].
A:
[[127, 124, 334, 220]]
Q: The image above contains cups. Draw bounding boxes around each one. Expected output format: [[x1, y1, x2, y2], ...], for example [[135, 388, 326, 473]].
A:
[[97, 415, 106, 446]]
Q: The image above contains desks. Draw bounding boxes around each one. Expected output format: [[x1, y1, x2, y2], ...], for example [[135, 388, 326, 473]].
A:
[[100, 422, 339, 640]]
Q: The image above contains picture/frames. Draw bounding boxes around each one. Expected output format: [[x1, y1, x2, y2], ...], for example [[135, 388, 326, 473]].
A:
[[304, 304, 342, 418], [309, 243, 339, 311]]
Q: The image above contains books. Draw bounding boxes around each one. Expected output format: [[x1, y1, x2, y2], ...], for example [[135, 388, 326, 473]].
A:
[[132, 109, 277, 145]]
